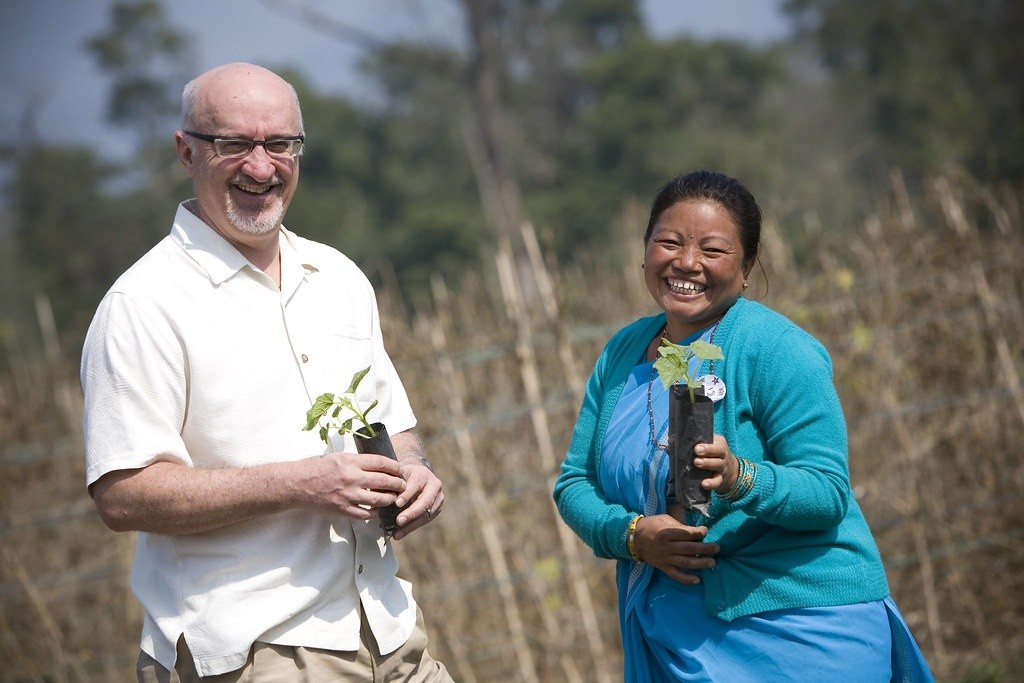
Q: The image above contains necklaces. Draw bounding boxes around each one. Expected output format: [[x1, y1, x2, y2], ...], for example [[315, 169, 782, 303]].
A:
[[648, 321, 720, 447]]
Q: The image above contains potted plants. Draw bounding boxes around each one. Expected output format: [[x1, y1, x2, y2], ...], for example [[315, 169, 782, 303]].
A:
[[302, 365, 412, 535], [652, 336, 724, 509]]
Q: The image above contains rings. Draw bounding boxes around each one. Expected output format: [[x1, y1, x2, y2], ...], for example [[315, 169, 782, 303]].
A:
[[427, 509, 433, 520]]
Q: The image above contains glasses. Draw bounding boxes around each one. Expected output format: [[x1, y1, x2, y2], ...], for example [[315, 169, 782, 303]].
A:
[[184, 129, 305, 160]]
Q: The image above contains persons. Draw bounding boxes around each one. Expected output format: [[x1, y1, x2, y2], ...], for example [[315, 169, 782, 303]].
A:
[[77, 63, 456, 683], [551, 170, 934, 683]]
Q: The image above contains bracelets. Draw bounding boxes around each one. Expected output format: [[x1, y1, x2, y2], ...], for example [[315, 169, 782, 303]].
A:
[[714, 456, 758, 502], [630, 514, 647, 565]]
